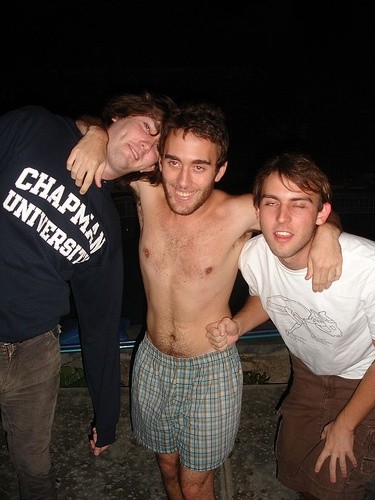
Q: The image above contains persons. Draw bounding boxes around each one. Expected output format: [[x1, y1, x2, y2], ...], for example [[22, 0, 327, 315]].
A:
[[1, 92, 177, 500], [211, 154, 375, 500], [67, 103, 341, 500]]
